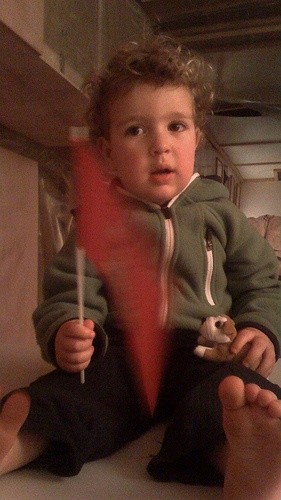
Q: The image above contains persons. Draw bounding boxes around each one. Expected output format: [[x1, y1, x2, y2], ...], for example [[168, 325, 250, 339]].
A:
[[0, 32, 281, 500]]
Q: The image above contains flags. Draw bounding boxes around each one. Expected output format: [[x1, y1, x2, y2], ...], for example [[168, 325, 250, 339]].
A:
[[77, 135, 167, 416]]
